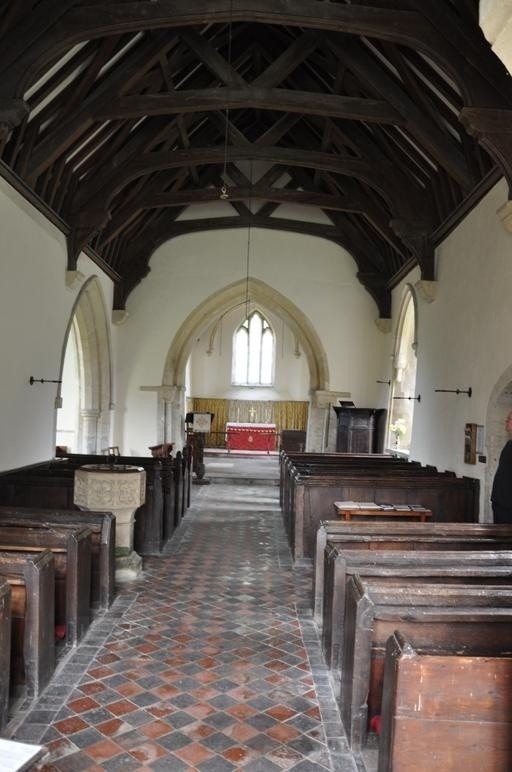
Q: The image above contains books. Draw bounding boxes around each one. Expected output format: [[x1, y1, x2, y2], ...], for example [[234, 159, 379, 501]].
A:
[[334, 499, 429, 512]]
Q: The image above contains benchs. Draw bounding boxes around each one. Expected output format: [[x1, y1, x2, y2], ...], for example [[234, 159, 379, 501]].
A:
[[1, 506, 117, 738], [276, 449, 481, 568], [1, 446, 190, 553], [310, 520, 511, 772]]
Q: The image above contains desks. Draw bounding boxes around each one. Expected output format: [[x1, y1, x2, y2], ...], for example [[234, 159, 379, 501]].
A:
[[332, 500, 434, 522]]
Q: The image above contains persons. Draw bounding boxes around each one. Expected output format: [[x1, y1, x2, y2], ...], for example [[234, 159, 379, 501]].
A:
[[490, 412, 512, 524]]
